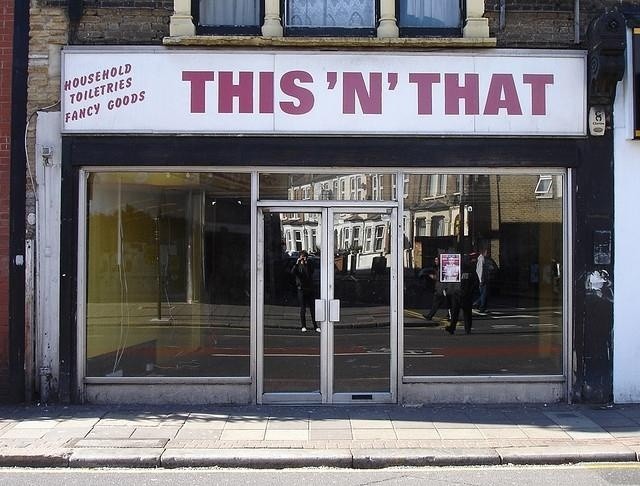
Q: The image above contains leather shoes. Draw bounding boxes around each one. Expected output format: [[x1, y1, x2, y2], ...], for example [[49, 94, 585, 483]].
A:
[[445, 326, 454, 334], [423, 314, 432, 320]]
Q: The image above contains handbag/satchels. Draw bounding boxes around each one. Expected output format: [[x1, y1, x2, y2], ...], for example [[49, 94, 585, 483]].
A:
[[482, 257, 499, 288]]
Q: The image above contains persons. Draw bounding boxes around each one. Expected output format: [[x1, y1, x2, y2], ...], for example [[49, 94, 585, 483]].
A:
[[422, 248, 496, 335], [290, 251, 321, 333], [551, 258, 563, 296]]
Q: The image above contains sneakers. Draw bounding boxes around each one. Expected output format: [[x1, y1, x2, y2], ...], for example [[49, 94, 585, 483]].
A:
[[316, 328, 321, 333], [301, 328, 307, 332]]
